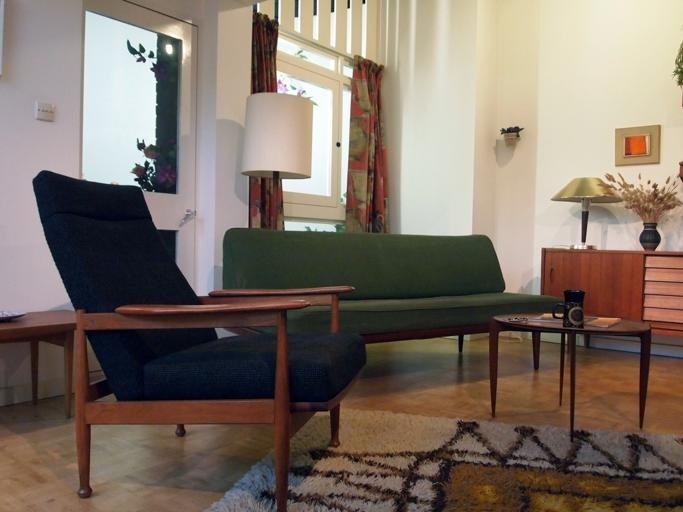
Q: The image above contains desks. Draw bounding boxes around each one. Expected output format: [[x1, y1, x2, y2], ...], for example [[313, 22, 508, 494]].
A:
[[1, 310, 83, 421]]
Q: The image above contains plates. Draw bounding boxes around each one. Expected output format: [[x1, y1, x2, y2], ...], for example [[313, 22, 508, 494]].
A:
[[1, 310, 26, 322]]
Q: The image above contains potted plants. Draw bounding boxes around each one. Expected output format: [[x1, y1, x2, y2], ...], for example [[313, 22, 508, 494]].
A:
[[500, 126, 524, 146]]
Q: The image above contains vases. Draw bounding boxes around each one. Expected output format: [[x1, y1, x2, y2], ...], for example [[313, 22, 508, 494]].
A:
[[638, 223, 661, 253]]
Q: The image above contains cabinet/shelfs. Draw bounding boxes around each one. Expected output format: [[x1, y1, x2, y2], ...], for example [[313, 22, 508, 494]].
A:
[[539, 245, 683, 343]]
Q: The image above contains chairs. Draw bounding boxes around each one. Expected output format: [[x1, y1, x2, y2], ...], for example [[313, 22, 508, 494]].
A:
[[25, 163, 365, 510]]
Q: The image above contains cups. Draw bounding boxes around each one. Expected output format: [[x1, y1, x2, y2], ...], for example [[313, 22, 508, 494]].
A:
[[552, 289, 585, 328]]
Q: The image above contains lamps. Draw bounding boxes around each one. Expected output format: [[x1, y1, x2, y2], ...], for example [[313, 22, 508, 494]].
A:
[[549, 176, 623, 250], [238, 92, 314, 228]]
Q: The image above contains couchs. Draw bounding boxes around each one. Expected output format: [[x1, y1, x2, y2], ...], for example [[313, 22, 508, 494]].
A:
[[221, 224, 562, 368]]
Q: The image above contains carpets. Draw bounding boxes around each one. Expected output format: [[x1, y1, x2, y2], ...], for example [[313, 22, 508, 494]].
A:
[[204, 405, 682, 511]]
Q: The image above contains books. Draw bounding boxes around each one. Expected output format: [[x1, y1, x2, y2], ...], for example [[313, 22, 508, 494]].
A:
[[528, 313, 622, 328]]
